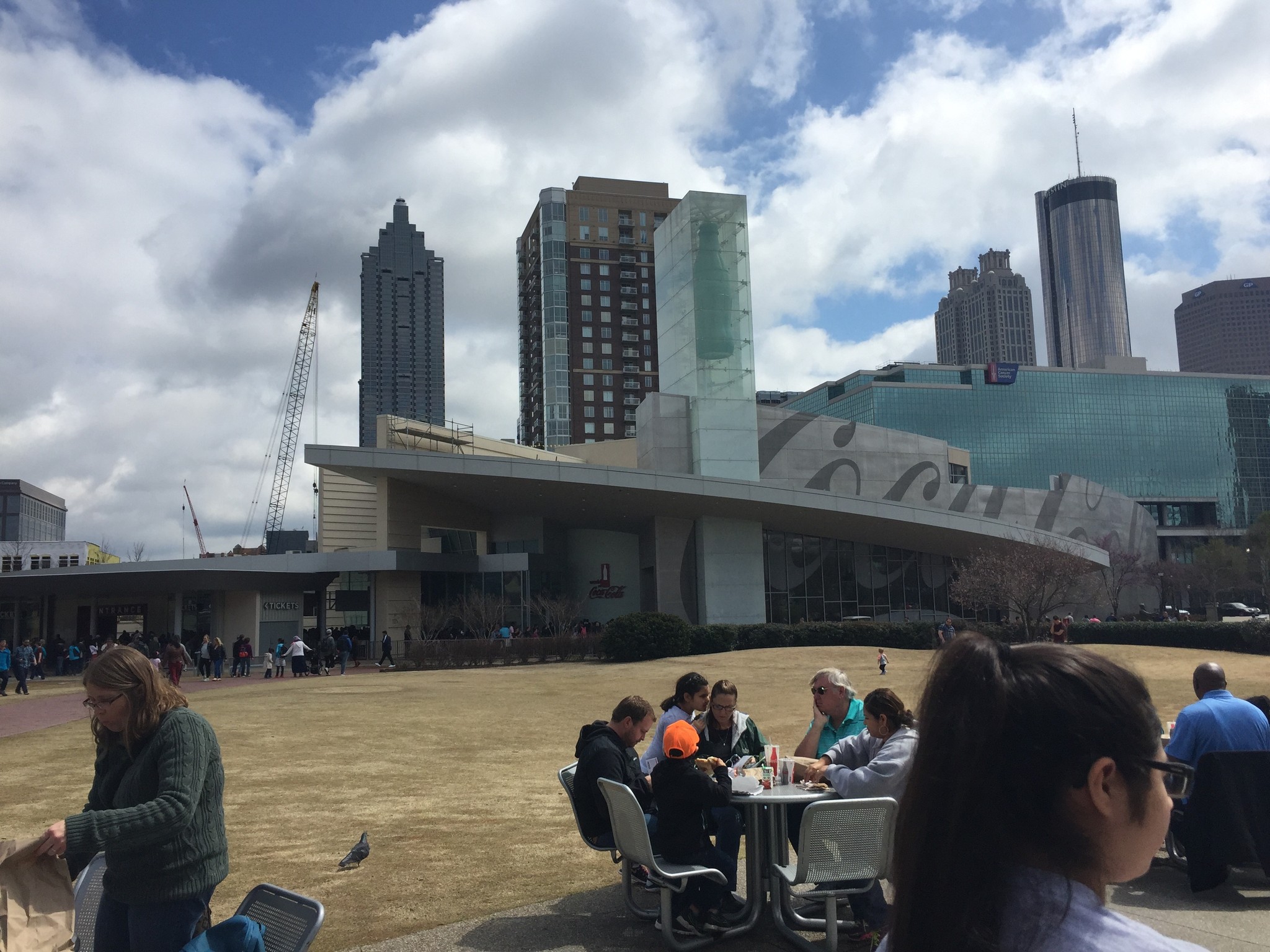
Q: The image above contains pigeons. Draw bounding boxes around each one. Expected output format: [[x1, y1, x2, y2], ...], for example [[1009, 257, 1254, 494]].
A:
[[338, 832, 370, 867]]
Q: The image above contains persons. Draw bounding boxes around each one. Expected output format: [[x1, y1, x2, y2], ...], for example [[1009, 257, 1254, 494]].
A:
[[1050, 615, 1066, 643], [263, 625, 370, 679], [375, 630, 396, 667], [26, 629, 227, 688], [1045, 616, 1051, 622], [1065, 612, 1074, 623], [938, 617, 956, 645], [1139, 603, 1190, 622], [997, 615, 1022, 626], [0, 637, 11, 696], [573, 667, 919, 942], [1163, 662, 1270, 891], [230, 634, 254, 678], [12, 638, 37, 695], [34, 645, 229, 952], [404, 625, 413, 659], [875, 632, 1209, 952], [1081, 612, 1137, 623], [877, 648, 889, 675], [422, 619, 610, 658]]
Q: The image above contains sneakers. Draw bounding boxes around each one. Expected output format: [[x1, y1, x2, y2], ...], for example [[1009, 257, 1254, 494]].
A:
[[212, 678, 217, 681], [203, 675, 209, 681], [375, 663, 381, 667], [847, 917, 890, 941], [216, 678, 222, 681], [388, 665, 395, 667], [618, 862, 748, 935]]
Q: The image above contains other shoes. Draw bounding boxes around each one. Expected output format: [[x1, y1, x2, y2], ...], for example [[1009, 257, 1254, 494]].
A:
[[0, 690, 8, 696], [341, 672, 346, 675], [24, 692, 30, 694], [16, 689, 22, 694], [26, 677, 33, 681], [324, 667, 331, 676], [803, 887, 849, 902]]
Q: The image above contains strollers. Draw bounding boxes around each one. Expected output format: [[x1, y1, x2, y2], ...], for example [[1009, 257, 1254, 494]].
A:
[[304, 646, 331, 676]]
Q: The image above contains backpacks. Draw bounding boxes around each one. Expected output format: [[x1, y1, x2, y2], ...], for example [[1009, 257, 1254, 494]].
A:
[[178, 914, 265, 952], [336, 636, 348, 651], [239, 644, 247, 657], [320, 637, 330, 652], [281, 645, 288, 658]]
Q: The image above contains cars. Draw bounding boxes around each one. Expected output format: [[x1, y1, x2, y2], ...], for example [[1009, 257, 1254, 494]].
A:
[[1220, 602, 1261, 616], [1165, 605, 1190, 616]]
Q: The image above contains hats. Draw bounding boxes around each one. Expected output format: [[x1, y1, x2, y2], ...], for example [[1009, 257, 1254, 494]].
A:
[[292, 635, 300, 641], [382, 631, 387, 633], [663, 720, 699, 759], [326, 629, 332, 634]]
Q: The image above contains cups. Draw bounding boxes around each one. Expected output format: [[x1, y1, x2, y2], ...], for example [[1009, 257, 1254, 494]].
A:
[[765, 745, 779, 780], [778, 758, 795, 785], [1167, 721, 1176, 731]]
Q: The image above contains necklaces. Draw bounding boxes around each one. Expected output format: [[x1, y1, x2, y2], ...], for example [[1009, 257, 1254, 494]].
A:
[[714, 726, 729, 746]]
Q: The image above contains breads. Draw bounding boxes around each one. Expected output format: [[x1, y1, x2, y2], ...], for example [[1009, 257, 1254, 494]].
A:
[[695, 756, 717, 774]]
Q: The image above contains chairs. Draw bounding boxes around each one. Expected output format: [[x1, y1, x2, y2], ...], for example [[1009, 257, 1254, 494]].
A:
[[557, 762, 899, 952], [233, 883, 323, 952], [1168, 751, 1270, 869], [72, 851, 107, 952]]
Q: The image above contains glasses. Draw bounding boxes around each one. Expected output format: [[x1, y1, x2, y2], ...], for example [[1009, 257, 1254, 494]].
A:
[[82, 682, 140, 710], [810, 685, 836, 694], [711, 700, 736, 712], [1122, 755, 1193, 799]]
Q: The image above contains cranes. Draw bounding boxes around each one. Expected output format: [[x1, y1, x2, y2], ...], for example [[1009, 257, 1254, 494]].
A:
[[181, 485, 209, 558], [234, 268, 322, 556]]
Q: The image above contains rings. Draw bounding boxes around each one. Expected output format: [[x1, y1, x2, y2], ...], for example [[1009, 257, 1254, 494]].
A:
[[50, 848, 56, 852], [817, 770, 819, 773]]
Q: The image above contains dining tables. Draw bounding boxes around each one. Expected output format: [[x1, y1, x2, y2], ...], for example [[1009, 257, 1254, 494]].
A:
[[679, 784, 837, 951]]
